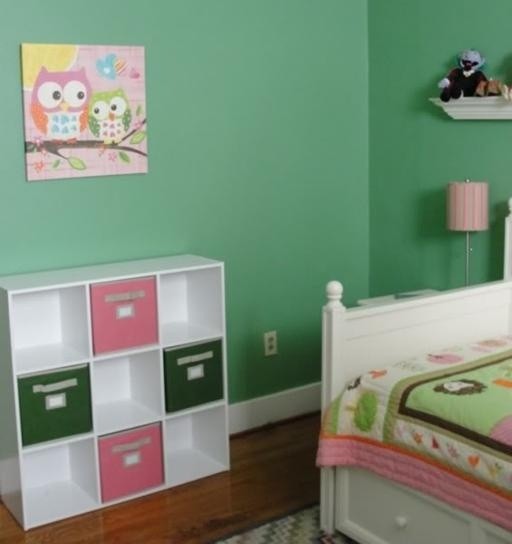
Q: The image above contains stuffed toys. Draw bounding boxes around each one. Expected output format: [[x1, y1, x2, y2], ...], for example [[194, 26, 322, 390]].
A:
[[434, 47, 488, 102]]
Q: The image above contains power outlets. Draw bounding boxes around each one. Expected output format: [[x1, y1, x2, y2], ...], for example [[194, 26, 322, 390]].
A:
[[265, 330, 279, 356]]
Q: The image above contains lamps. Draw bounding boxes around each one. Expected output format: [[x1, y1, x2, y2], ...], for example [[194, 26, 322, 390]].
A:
[[442, 174, 491, 287]]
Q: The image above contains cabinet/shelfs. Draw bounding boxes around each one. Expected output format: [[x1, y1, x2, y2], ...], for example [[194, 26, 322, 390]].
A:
[[0, 253, 232, 531]]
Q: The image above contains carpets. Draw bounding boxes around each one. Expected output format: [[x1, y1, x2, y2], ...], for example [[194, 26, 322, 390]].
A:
[[202, 491, 353, 542]]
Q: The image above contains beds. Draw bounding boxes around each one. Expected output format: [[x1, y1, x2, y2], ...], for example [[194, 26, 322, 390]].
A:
[[320, 268, 511, 542]]
